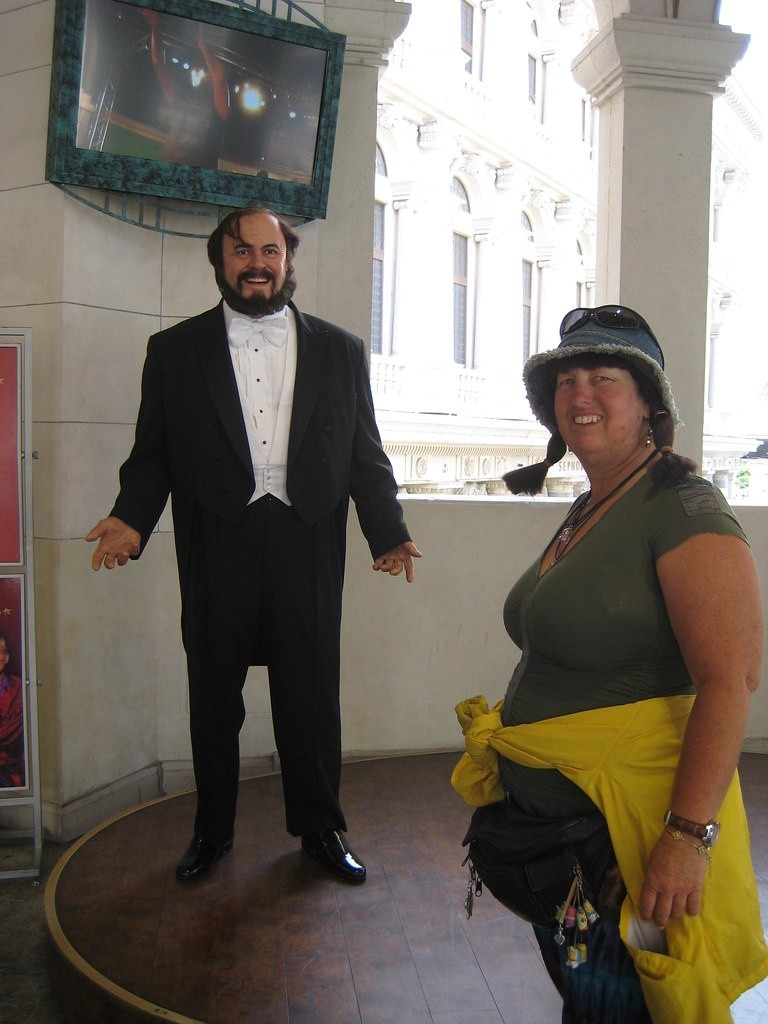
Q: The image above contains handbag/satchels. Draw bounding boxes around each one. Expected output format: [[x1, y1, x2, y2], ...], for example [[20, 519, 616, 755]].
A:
[[462, 800, 612, 925]]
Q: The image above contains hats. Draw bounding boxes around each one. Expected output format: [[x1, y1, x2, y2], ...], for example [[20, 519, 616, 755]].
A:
[[522, 312, 685, 433]]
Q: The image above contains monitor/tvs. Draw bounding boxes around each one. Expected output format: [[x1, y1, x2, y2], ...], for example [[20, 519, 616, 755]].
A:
[[44, 0, 348, 219]]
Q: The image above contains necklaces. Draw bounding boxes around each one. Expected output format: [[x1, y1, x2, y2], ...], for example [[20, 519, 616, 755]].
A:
[[545, 448, 661, 570]]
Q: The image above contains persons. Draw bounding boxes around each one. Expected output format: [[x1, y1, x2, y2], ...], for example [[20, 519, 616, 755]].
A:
[[86, 204, 423, 889], [449, 304, 767, 1024]]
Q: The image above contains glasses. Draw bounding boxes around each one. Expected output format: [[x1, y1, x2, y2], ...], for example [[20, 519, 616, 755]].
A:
[[560, 305, 664, 370]]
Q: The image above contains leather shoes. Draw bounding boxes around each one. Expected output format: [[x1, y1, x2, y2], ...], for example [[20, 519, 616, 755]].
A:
[[302, 829, 366, 884], [176, 827, 235, 882]]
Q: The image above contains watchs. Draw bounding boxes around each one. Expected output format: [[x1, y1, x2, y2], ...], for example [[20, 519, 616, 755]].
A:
[[665, 808, 722, 847]]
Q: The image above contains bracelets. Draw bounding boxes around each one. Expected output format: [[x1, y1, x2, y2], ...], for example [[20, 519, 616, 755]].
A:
[[660, 828, 714, 863]]
